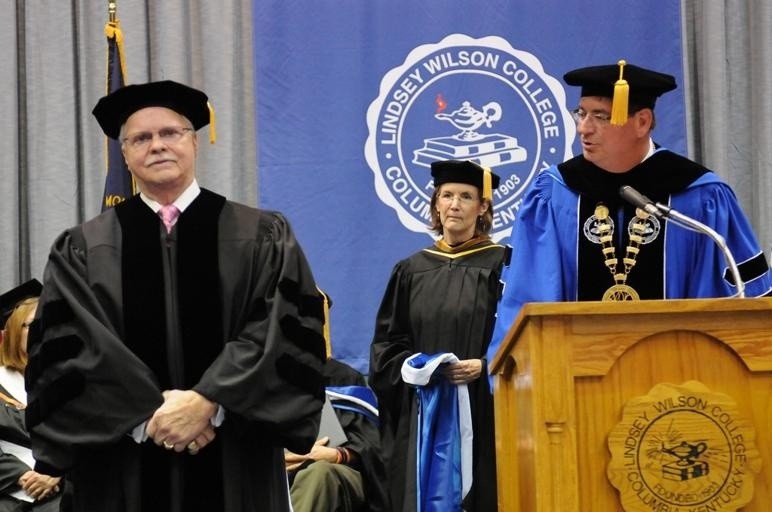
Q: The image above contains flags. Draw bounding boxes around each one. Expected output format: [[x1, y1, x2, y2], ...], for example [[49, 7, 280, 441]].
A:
[[100, 23, 138, 216]]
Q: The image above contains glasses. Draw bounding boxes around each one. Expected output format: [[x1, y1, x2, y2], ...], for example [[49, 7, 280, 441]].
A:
[[117, 126, 193, 145], [573, 108, 614, 127]]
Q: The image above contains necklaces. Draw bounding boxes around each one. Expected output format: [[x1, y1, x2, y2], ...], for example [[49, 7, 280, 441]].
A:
[[595, 200, 654, 302]]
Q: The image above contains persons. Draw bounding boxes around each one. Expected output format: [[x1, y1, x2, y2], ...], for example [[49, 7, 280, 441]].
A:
[[280, 361, 379, 512], [486, 61, 772, 395], [369, 159, 511, 511], [0, 277, 64, 512], [25, 81, 322, 512]]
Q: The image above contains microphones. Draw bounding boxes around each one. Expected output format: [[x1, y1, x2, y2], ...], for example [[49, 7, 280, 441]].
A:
[[618, 183, 746, 297]]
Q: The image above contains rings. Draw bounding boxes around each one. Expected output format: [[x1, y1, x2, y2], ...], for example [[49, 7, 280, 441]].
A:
[[188, 442, 198, 454], [162, 441, 174, 450]]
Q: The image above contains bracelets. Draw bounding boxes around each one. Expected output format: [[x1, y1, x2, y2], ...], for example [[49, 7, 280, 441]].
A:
[[334, 447, 352, 466]]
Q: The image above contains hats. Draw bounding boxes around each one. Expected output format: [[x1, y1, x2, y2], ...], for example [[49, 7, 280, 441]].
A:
[[93, 81, 216, 144], [0, 278, 43, 331], [562, 61, 677, 130], [431, 161, 501, 204]]
